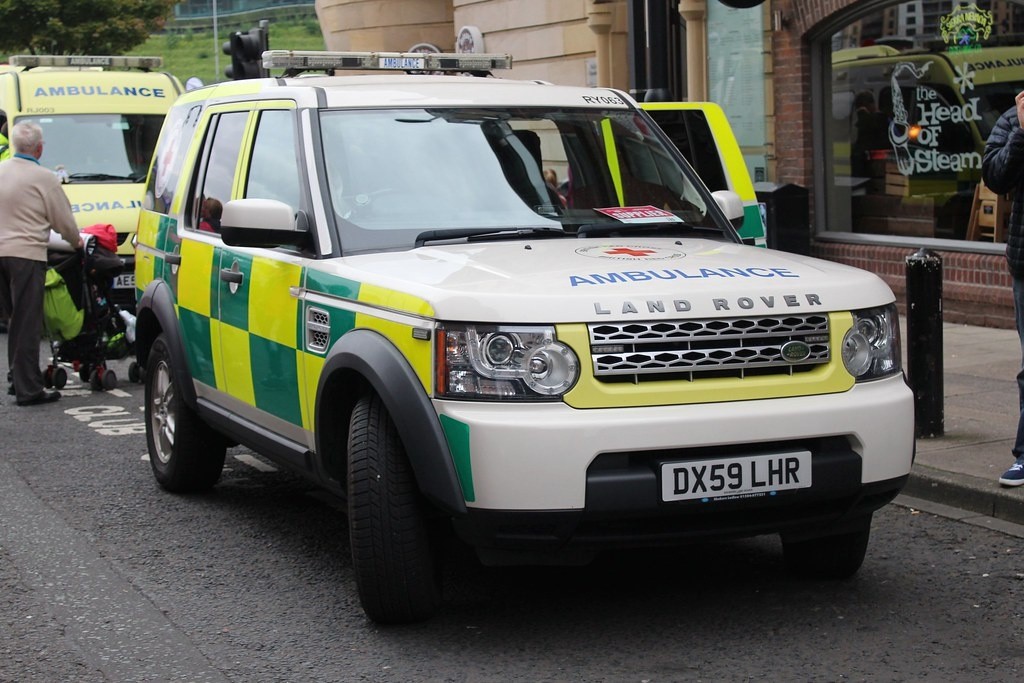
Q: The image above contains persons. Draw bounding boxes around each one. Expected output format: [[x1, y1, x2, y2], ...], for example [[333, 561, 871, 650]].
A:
[[197, 197, 222, 235], [981, 91, 1024, 488], [0, 120, 84, 406]]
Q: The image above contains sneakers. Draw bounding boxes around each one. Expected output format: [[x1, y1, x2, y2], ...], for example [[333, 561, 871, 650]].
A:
[[998, 463, 1024, 486]]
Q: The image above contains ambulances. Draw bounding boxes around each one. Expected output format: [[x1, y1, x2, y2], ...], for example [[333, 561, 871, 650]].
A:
[[828, 40, 1024, 206], [1, 55, 193, 316], [129, 47, 917, 630]]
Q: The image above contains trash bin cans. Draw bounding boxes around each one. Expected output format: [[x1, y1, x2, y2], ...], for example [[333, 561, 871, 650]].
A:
[[835, 176, 874, 234], [753, 181, 811, 257]]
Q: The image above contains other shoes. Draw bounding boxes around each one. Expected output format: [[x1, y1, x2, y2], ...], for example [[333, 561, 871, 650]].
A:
[[16, 391, 61, 406], [6, 383, 16, 395]]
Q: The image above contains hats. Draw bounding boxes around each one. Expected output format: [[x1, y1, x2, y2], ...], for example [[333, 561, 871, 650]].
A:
[[851, 90, 874, 106]]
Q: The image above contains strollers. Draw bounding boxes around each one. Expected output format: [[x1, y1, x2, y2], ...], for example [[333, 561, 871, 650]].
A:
[[45, 247, 139, 391]]
